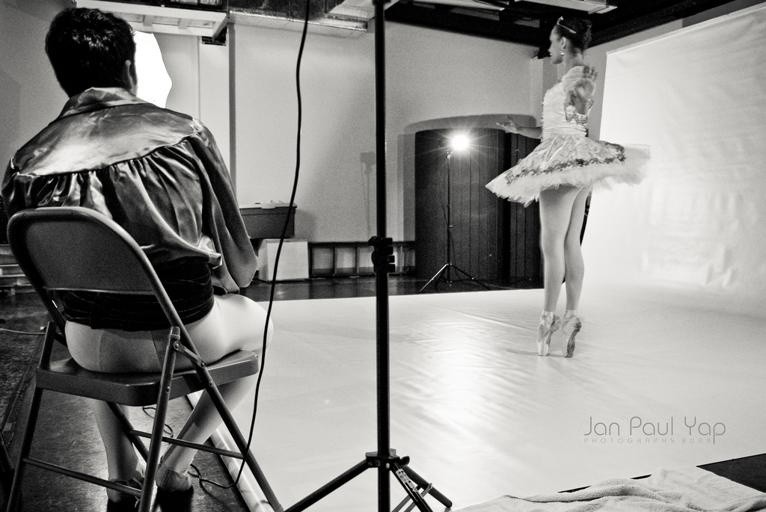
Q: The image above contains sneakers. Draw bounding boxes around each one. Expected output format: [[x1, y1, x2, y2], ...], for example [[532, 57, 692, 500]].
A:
[[154, 457, 192, 491], [106, 467, 145, 503], [537, 314, 561, 356], [562, 314, 582, 357]]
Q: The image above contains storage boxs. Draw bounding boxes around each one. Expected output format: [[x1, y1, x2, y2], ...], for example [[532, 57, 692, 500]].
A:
[[240, 203, 297, 240], [257, 238, 310, 282]]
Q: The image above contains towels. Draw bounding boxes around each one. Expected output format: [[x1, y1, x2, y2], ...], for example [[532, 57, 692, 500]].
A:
[[452, 466, 766, 512]]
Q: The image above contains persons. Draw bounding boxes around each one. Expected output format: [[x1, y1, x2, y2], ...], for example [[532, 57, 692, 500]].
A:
[[1, 7, 271, 510], [482, 15, 653, 363]]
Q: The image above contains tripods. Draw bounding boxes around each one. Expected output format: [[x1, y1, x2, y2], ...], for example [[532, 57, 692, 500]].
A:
[[283, 0, 452, 512], [415, 159, 492, 295]]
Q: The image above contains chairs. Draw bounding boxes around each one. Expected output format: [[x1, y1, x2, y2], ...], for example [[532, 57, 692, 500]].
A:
[[7, 207, 284, 512]]
